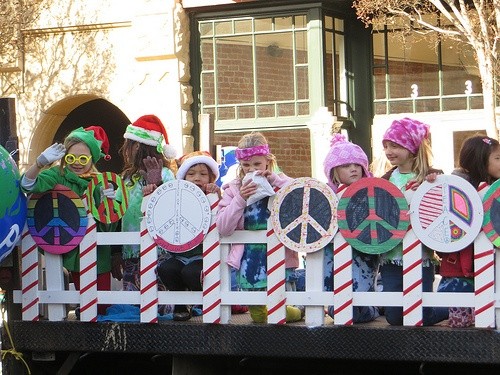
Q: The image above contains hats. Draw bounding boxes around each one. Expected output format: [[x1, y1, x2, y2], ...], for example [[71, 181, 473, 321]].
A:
[[64, 126, 111, 164], [123, 115, 177, 159], [175, 151, 220, 183], [324, 133, 373, 193]]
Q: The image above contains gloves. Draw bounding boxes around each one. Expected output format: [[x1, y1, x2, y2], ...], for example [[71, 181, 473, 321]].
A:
[[36, 143, 67, 168], [141, 156, 163, 185], [100, 183, 116, 203], [111, 253, 126, 281]]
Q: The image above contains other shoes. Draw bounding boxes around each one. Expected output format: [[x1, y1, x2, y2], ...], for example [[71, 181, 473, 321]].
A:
[[173, 305, 193, 321]]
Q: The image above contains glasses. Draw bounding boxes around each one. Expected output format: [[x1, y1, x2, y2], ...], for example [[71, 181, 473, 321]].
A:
[[64, 154, 92, 165]]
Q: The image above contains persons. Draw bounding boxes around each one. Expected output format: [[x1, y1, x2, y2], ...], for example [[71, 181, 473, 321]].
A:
[[218, 132, 305, 325], [116, 115, 171, 315], [437, 136, 500, 326], [20, 126, 115, 318], [323, 134, 380, 322], [378, 118, 450, 325], [142, 151, 221, 319]]
